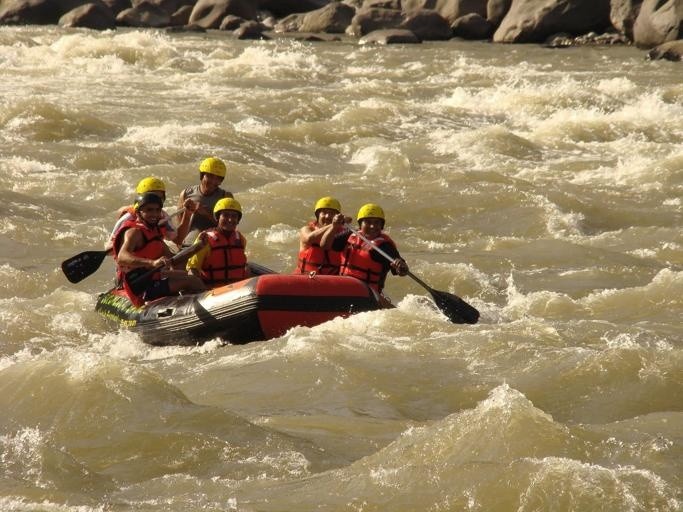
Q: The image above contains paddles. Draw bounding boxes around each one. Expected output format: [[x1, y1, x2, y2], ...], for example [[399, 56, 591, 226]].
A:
[[62, 204, 187, 283], [125, 239, 204, 295], [342, 220, 481, 324]]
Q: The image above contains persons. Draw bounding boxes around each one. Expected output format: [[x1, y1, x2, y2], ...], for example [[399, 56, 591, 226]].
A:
[[174, 157, 235, 250], [109, 193, 210, 305], [102, 175, 197, 291], [318, 202, 409, 297], [293, 195, 354, 278], [187, 196, 256, 293]]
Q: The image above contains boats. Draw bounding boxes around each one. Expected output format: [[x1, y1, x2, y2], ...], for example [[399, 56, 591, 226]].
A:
[[92, 258, 396, 349]]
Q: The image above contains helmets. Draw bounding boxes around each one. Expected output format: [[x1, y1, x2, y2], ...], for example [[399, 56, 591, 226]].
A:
[[200, 158, 226, 181], [133, 178, 165, 214], [315, 197, 341, 216], [214, 198, 242, 223], [357, 204, 385, 229]]
